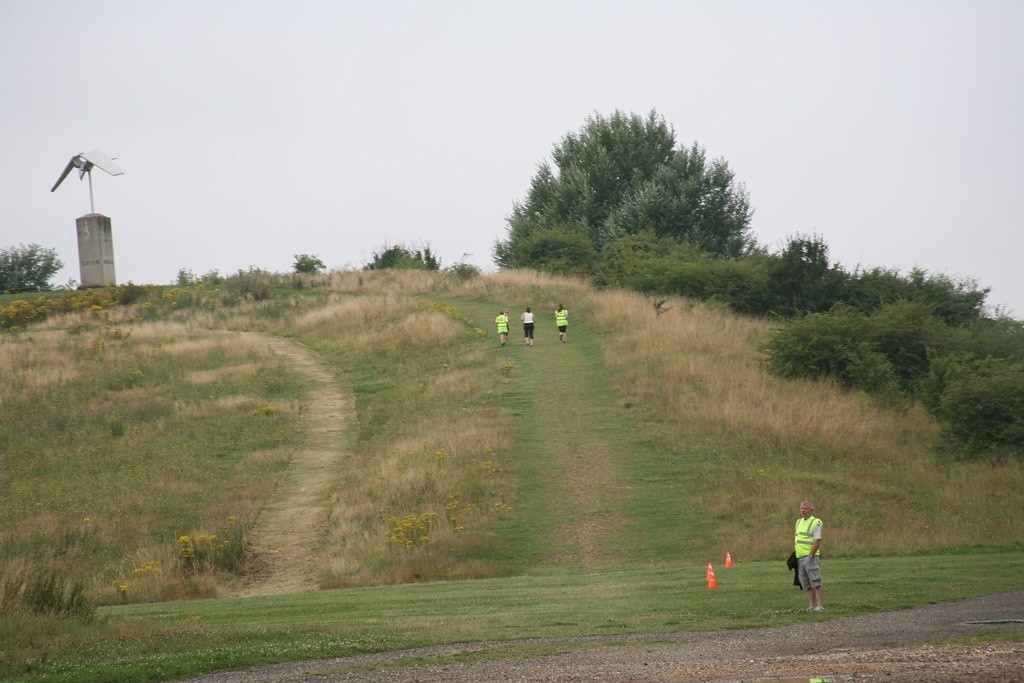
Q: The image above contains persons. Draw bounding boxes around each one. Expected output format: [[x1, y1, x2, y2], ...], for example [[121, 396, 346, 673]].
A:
[[794, 501, 824, 612], [554, 304, 569, 342], [521, 307, 535, 346], [495, 308, 512, 346]]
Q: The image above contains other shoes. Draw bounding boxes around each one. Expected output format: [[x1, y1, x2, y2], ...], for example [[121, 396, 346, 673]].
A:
[[806, 607, 814, 611], [814, 604, 824, 611]]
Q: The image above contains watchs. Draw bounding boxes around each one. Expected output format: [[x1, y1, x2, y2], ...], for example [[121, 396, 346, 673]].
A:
[[810, 555, 813, 557]]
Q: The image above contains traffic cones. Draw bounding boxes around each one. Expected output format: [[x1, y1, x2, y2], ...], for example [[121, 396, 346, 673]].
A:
[[725, 552, 733, 567], [708, 568, 717, 589], [705, 562, 715, 580]]
